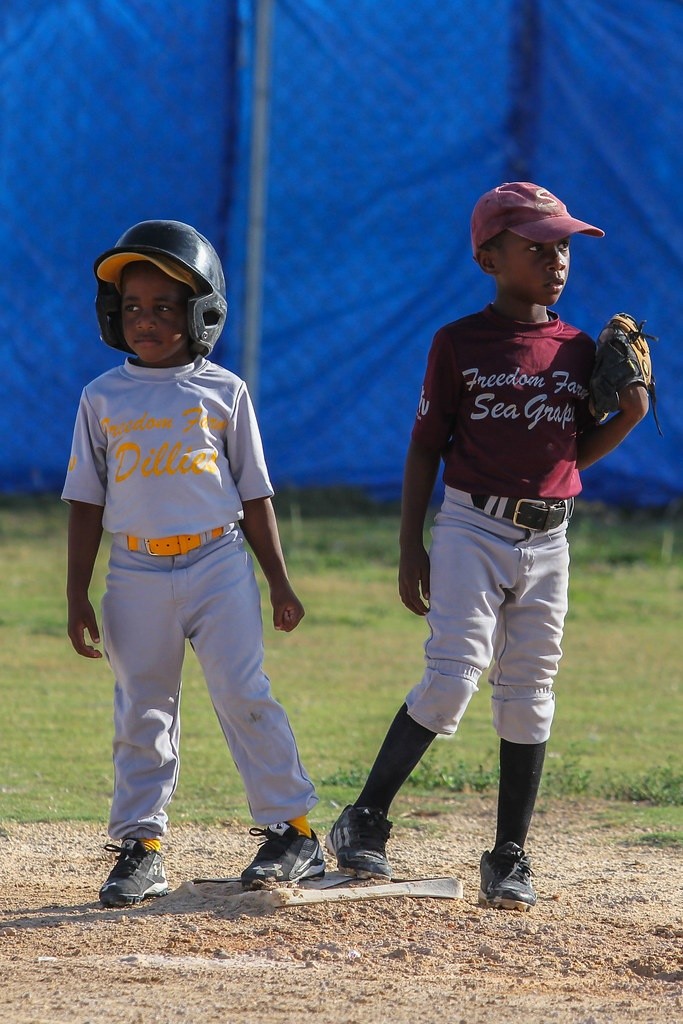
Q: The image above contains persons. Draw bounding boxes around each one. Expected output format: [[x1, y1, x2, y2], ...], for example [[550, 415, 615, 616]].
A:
[[58, 216, 327, 909], [324, 180, 651, 912]]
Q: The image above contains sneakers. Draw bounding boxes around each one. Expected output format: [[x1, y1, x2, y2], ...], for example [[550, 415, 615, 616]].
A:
[[98, 838, 169, 907], [326, 805, 392, 881], [478, 842, 537, 912], [242, 820, 326, 891]]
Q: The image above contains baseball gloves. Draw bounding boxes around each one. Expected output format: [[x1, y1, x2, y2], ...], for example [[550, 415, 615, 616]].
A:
[[590, 312, 666, 440]]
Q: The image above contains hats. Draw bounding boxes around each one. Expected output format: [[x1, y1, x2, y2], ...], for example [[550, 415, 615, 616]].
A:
[[96, 252, 204, 296], [470, 182, 605, 262]]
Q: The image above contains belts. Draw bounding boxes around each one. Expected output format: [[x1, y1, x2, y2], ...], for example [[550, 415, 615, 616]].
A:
[[111, 522, 235, 557], [471, 493, 573, 533]]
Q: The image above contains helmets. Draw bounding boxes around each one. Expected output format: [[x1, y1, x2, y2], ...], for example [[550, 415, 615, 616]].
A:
[[93, 220, 228, 358]]
